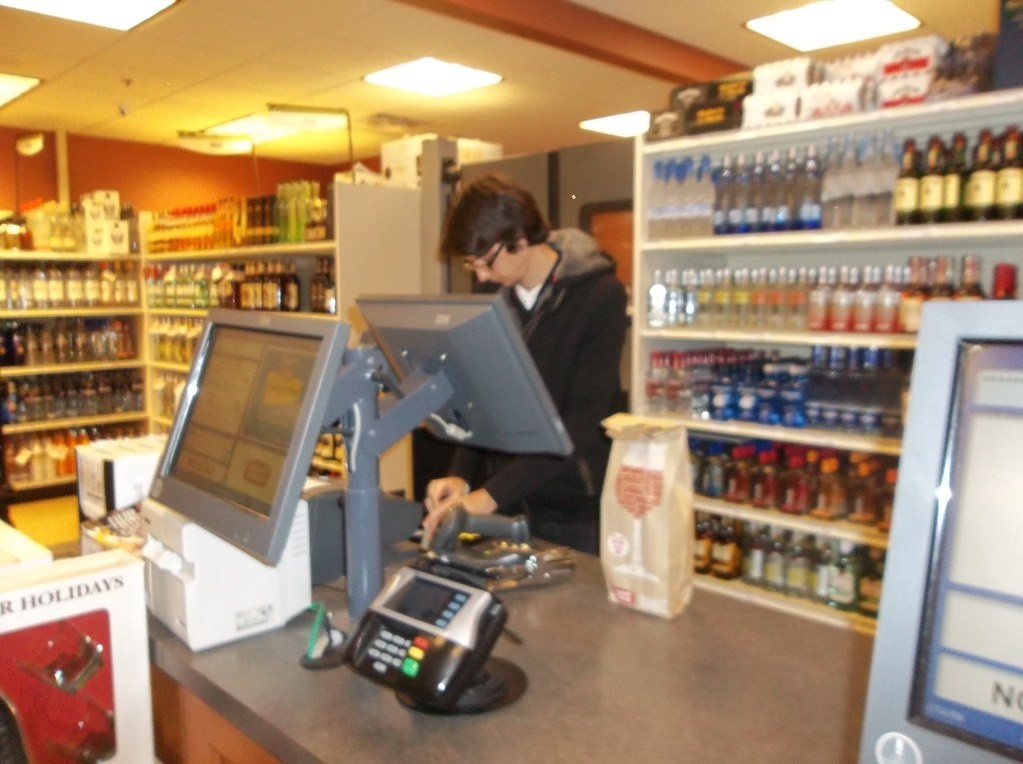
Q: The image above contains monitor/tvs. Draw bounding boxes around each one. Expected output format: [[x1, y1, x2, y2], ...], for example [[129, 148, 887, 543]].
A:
[[148, 308, 350, 567], [857, 300, 1023, 764], [356, 293, 574, 455]]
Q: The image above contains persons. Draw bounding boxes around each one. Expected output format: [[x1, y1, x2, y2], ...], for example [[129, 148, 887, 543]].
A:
[[423, 177, 627, 554]]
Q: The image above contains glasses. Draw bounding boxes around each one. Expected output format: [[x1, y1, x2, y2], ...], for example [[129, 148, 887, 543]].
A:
[[463, 238, 508, 274]]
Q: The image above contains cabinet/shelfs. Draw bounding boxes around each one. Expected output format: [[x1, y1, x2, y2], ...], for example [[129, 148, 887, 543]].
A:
[[628, 85, 1023, 632], [1, 244, 345, 499]]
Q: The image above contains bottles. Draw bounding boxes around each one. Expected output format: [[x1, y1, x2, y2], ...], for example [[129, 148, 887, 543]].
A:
[[150, 204, 217, 254], [1, 369, 145, 424], [148, 316, 203, 367], [800, 30, 997, 120], [310, 257, 337, 315], [1, 424, 148, 485], [694, 513, 888, 620], [0, 261, 139, 311], [216, 196, 276, 250], [1, 316, 136, 368], [121, 204, 139, 252], [0, 215, 19, 252], [308, 419, 346, 482], [18, 194, 87, 255], [144, 258, 301, 312], [277, 179, 334, 243], [153, 370, 187, 422], [645, 126, 1023, 240], [689, 436, 898, 532], [648, 255, 1017, 334], [646, 345, 915, 440], [91, 194, 124, 246]]
